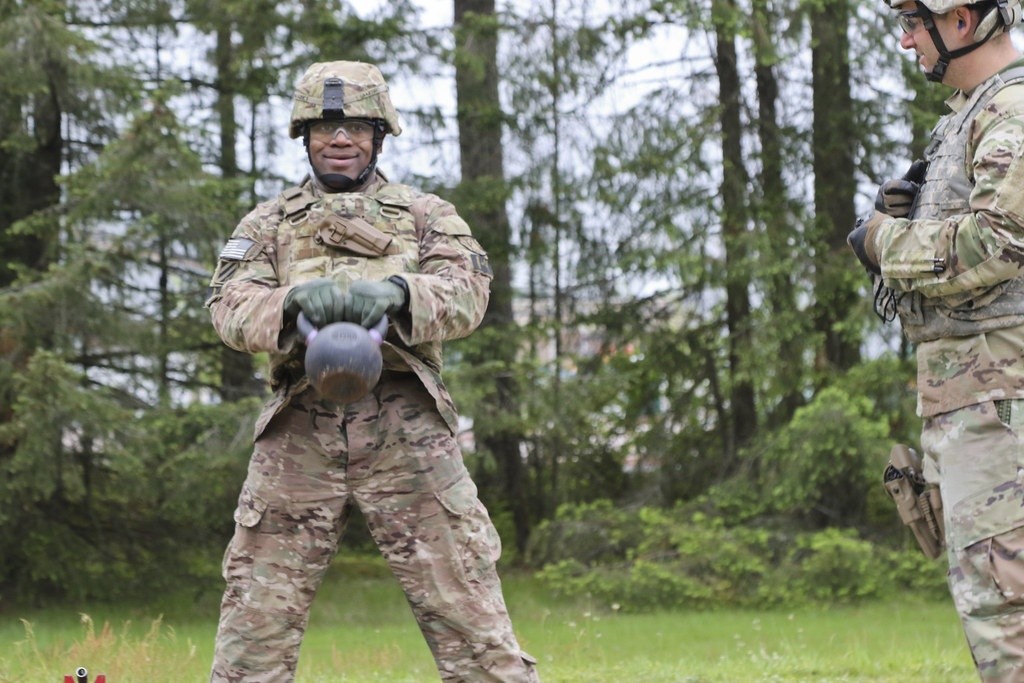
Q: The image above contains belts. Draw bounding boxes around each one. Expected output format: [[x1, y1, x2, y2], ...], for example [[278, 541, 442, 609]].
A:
[[378, 369, 417, 385]]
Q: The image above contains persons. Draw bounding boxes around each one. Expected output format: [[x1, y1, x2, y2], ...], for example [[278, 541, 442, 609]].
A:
[[208, 61, 537, 683], [847, 0, 1024, 682]]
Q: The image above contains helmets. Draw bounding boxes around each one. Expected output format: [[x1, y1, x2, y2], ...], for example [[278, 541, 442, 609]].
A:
[[290, 60, 402, 140], [882, 0, 1022, 43]]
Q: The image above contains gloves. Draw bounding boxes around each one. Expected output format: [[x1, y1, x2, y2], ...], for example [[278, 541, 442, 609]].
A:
[[346, 280, 405, 331], [283, 277, 345, 330], [875, 159, 927, 217], [847, 216, 881, 275]]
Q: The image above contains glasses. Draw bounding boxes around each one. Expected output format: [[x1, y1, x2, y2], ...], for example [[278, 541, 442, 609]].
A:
[[309, 122, 373, 139], [895, 10, 936, 36]]
